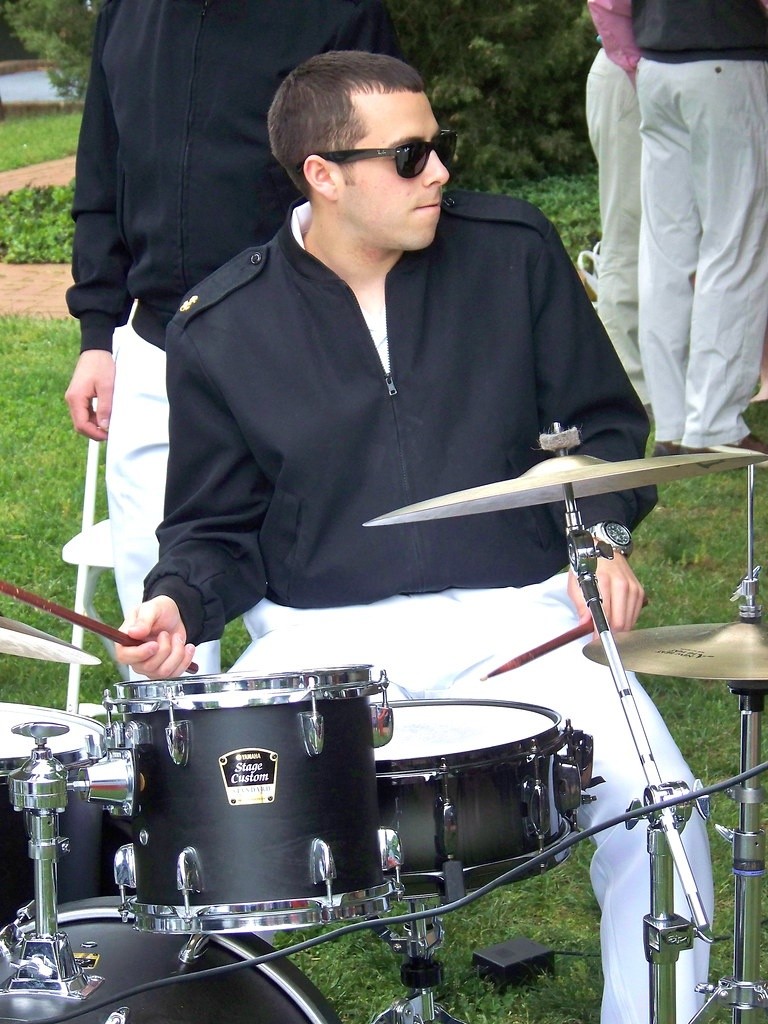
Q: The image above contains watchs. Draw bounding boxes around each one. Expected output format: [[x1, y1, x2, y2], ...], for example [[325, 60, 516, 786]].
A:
[[586, 521, 634, 561]]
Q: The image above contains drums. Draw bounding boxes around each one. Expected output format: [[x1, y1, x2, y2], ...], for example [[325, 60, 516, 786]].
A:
[[0, 895, 344, 1024], [113, 660, 405, 936], [0, 699, 106, 930], [368, 697, 607, 900]]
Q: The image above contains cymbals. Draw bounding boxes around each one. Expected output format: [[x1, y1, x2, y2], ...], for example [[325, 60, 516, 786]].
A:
[[0, 613, 103, 668], [362, 446, 768, 528], [582, 619, 767, 683]]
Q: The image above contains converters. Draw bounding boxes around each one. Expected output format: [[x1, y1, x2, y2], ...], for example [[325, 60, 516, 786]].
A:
[[471, 936, 555, 997]]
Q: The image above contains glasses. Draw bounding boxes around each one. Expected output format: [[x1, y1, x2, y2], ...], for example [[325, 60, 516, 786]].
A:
[[295, 129, 458, 178]]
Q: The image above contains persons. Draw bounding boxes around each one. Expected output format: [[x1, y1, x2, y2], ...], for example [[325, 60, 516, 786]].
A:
[[64, 0, 403, 684], [112, 52, 712, 1024], [586, 1, 767, 470]]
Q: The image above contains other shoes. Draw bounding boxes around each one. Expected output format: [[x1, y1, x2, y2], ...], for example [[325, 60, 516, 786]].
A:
[[651, 434, 768, 457]]
